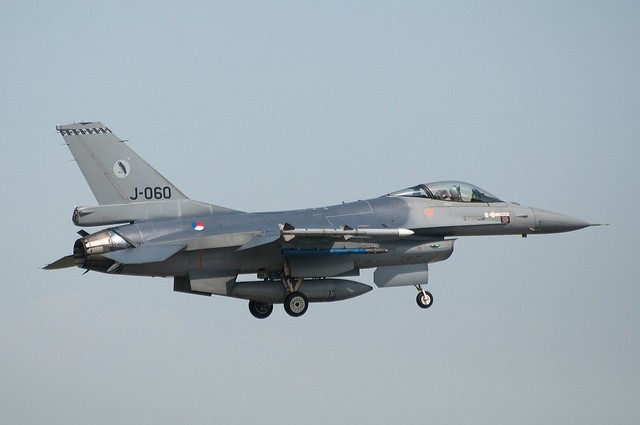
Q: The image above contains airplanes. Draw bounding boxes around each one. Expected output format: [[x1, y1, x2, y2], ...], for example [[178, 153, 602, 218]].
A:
[[42, 121, 611, 319]]
